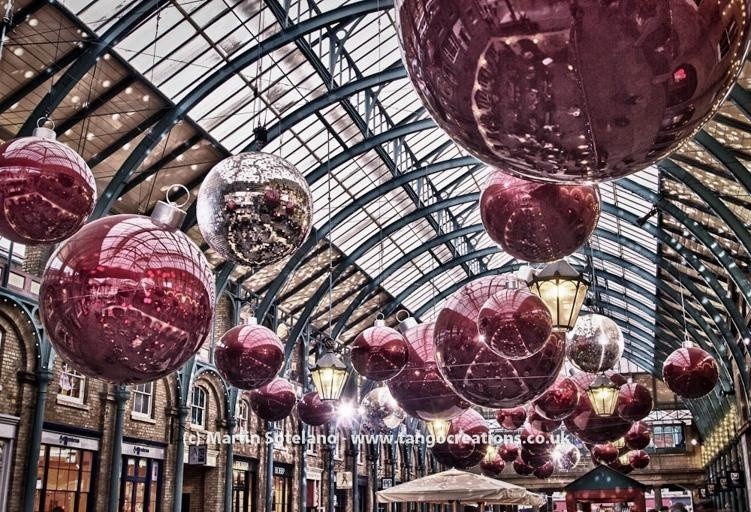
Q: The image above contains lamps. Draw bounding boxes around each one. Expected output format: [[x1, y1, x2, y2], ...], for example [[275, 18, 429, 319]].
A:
[[586, 369, 621, 417], [525, 257, 591, 331], [423, 173, 453, 443], [308, 126, 349, 400]]
